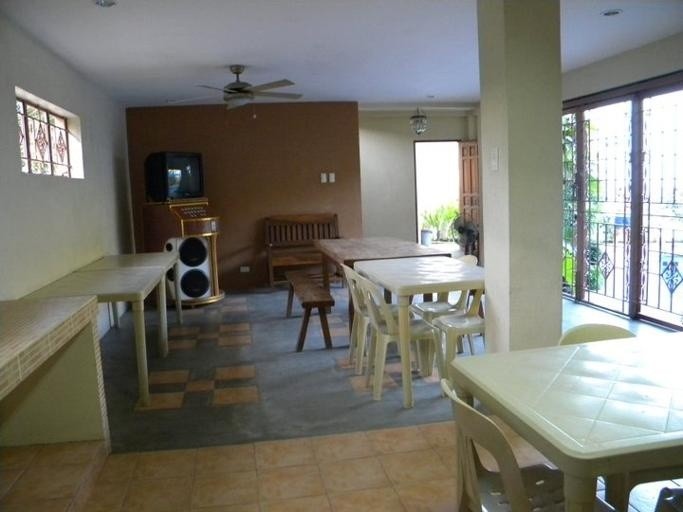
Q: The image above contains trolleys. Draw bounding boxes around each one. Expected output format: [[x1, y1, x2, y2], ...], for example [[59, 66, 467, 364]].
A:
[[451, 213, 479, 255], [195, 65, 303, 111]]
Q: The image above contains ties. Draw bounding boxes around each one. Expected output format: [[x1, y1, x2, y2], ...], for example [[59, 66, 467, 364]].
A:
[[143, 152, 205, 202]]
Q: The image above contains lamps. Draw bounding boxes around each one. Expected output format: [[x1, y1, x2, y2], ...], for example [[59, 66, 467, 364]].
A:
[[559, 323, 683, 512], [340, 254, 485, 409], [441, 378, 618, 512]]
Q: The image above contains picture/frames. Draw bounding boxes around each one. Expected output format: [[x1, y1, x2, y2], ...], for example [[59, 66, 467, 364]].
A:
[[73, 251, 183, 354], [16, 268, 168, 406]]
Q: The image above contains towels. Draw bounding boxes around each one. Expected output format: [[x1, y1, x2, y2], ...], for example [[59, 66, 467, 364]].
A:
[[421, 230, 432, 246]]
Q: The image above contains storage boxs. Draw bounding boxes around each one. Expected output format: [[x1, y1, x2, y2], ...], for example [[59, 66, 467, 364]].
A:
[[284, 269, 334, 352], [263, 213, 345, 288]]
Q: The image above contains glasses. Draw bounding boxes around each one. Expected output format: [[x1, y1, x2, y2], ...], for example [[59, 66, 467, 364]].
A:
[[224, 92, 253, 106], [409, 105, 427, 135]]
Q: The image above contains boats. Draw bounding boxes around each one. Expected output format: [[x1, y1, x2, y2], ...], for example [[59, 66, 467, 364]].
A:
[[161, 238, 213, 302]]
